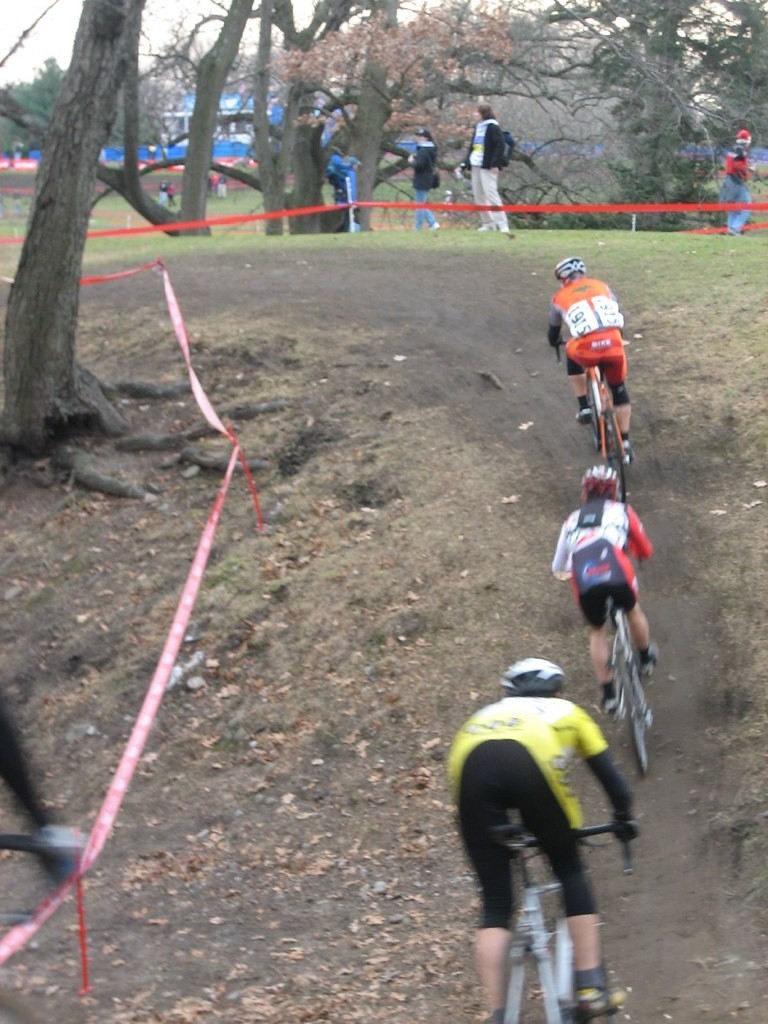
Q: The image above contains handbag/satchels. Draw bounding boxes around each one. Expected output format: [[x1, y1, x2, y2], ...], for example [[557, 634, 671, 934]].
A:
[[429, 174, 440, 188]]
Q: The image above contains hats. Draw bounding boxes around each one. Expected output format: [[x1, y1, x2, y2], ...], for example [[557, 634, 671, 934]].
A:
[[348, 157, 361, 164], [735, 130, 751, 143], [416, 130, 433, 138]]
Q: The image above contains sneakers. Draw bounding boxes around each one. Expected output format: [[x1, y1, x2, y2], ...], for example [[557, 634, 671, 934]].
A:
[[577, 988, 627, 1017]]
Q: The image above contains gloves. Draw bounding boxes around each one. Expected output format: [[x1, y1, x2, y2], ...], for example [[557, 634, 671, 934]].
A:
[[550, 336, 563, 346], [609, 811, 638, 840]]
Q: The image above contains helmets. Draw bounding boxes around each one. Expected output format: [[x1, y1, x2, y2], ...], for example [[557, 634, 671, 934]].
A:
[[555, 257, 587, 278], [500, 658, 565, 696], [579, 464, 620, 502]]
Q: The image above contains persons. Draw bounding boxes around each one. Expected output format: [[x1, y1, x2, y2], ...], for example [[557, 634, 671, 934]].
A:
[[455, 104, 509, 232], [159, 180, 177, 207], [408, 130, 441, 230], [325, 147, 362, 231], [547, 256, 633, 463], [552, 465, 660, 714], [449, 657, 634, 1024], [209, 172, 227, 198], [726, 130, 756, 236], [0, 703, 88, 875]]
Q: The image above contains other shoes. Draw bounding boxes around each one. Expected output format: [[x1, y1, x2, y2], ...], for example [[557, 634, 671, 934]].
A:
[[576, 408, 593, 425], [600, 694, 619, 712], [476, 224, 494, 231], [618, 440, 630, 464], [428, 222, 440, 231], [637, 641, 661, 677], [500, 225, 509, 233]]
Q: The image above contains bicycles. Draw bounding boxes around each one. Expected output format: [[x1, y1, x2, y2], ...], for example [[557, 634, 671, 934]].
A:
[[477, 819, 639, 1024], [554, 335, 631, 502], [603, 594, 654, 777]]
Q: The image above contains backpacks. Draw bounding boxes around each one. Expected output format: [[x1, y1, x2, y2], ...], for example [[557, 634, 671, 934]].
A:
[[501, 130, 513, 166]]
